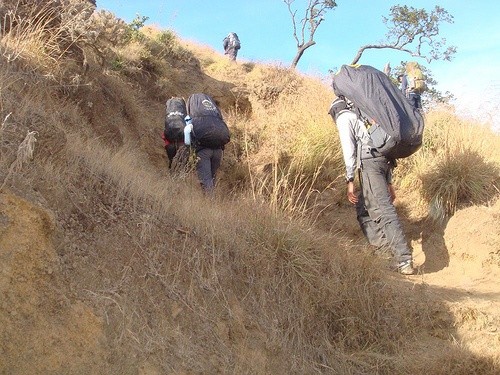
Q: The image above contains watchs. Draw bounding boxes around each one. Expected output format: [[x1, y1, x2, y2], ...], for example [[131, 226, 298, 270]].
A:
[[346, 178, 353, 184]]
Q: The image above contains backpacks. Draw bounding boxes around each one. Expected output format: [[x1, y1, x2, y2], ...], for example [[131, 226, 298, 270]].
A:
[[227, 32, 240, 55], [406, 62, 426, 93], [165, 98, 187, 141], [333, 64, 424, 159], [187, 94, 230, 153]]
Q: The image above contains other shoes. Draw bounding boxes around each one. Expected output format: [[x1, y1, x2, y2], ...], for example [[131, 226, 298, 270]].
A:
[[373, 247, 394, 257], [391, 259, 414, 274]]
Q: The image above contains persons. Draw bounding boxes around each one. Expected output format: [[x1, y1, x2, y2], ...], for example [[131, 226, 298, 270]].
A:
[[223, 37, 238, 61], [184, 94, 231, 196], [162, 96, 186, 170], [397, 75, 427, 120], [328, 94, 414, 274]]
